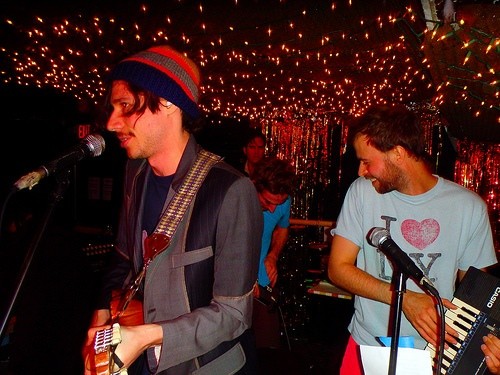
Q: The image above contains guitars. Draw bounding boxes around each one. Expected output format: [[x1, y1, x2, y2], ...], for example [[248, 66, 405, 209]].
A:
[[87, 287, 145, 375]]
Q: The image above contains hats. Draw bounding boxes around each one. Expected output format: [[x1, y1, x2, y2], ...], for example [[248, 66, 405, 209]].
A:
[[110, 46, 200, 120]]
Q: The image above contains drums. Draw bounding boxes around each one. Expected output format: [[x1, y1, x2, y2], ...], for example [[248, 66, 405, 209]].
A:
[[252, 285, 282, 353]]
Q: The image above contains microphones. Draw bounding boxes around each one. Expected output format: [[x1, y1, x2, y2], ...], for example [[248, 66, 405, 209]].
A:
[[368, 226, 439, 296], [13, 134, 106, 191]]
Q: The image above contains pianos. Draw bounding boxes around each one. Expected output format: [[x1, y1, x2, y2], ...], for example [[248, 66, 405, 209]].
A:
[[423, 264, 500, 375]]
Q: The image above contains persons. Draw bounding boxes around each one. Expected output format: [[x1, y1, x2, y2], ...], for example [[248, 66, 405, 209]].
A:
[[328, 107, 500, 375], [105, 44, 263, 375], [233, 132, 266, 177], [250, 157, 295, 308]]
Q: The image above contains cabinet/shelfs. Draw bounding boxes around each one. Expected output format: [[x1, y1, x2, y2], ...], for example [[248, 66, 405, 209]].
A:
[[76, 160, 116, 225]]
